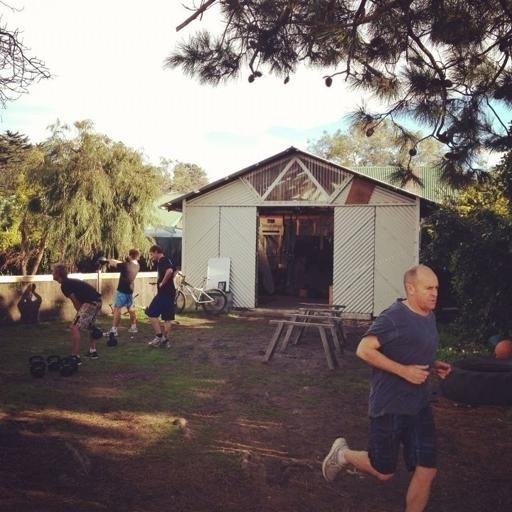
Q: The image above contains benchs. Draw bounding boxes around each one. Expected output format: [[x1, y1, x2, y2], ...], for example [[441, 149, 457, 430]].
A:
[[262, 303, 347, 371]]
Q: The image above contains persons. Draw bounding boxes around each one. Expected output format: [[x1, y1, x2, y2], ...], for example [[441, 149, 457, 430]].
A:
[[102, 249, 141, 337], [320, 264, 453, 511], [52, 264, 102, 366], [144, 245, 178, 349]]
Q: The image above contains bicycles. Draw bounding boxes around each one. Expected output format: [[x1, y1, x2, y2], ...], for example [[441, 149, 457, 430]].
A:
[[173, 271, 227, 318]]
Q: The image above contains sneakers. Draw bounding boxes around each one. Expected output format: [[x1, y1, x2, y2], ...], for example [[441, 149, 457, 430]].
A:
[[161, 335, 168, 344], [69, 355, 81, 366], [128, 327, 138, 332], [102, 331, 117, 337], [322, 438, 349, 483], [85, 351, 97, 359], [148, 337, 160, 347]]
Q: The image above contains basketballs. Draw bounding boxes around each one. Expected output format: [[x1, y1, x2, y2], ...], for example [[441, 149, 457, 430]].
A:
[[495, 341, 512, 360]]
